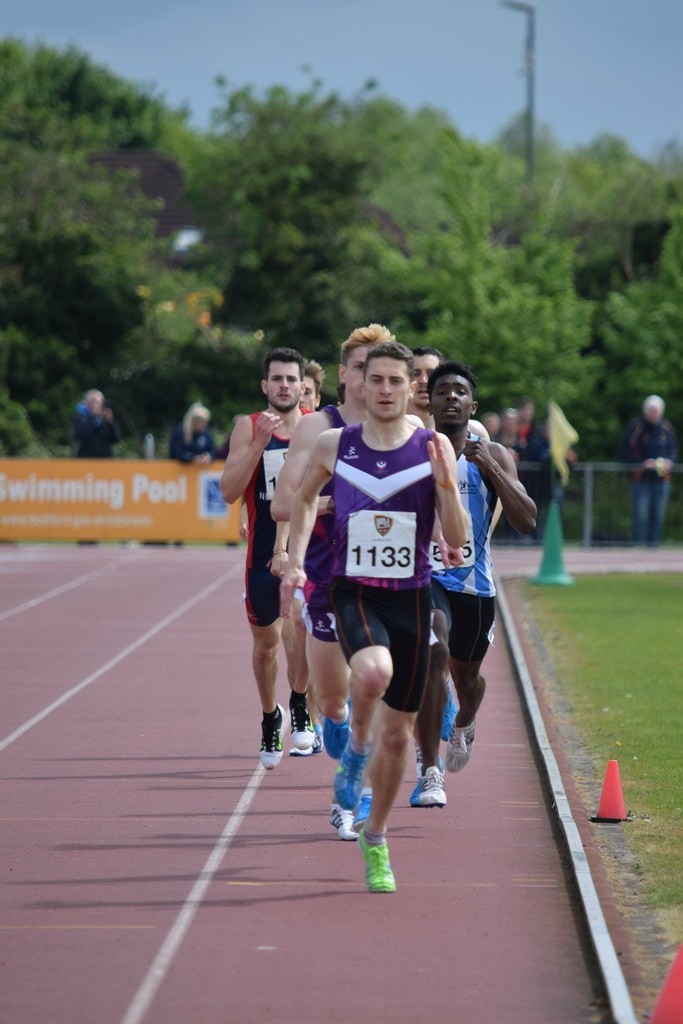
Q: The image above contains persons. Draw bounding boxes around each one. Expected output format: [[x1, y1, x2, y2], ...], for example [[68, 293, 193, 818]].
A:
[[168, 403, 218, 467], [72, 389, 124, 459], [282, 339, 475, 895], [220, 324, 538, 839], [480, 395, 576, 548], [625, 394, 679, 547]]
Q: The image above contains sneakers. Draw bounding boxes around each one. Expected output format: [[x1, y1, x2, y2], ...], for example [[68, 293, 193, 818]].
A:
[[290, 721, 322, 757], [289, 692, 314, 749], [441, 684, 458, 741], [351, 795, 372, 830], [330, 801, 360, 841], [358, 827, 396, 894], [410, 739, 448, 809], [334, 736, 371, 809], [447, 710, 475, 773], [322, 697, 353, 760], [260, 704, 285, 770]]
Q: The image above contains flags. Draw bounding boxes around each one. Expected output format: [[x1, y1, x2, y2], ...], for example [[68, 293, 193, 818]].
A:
[[548, 401, 579, 486]]
